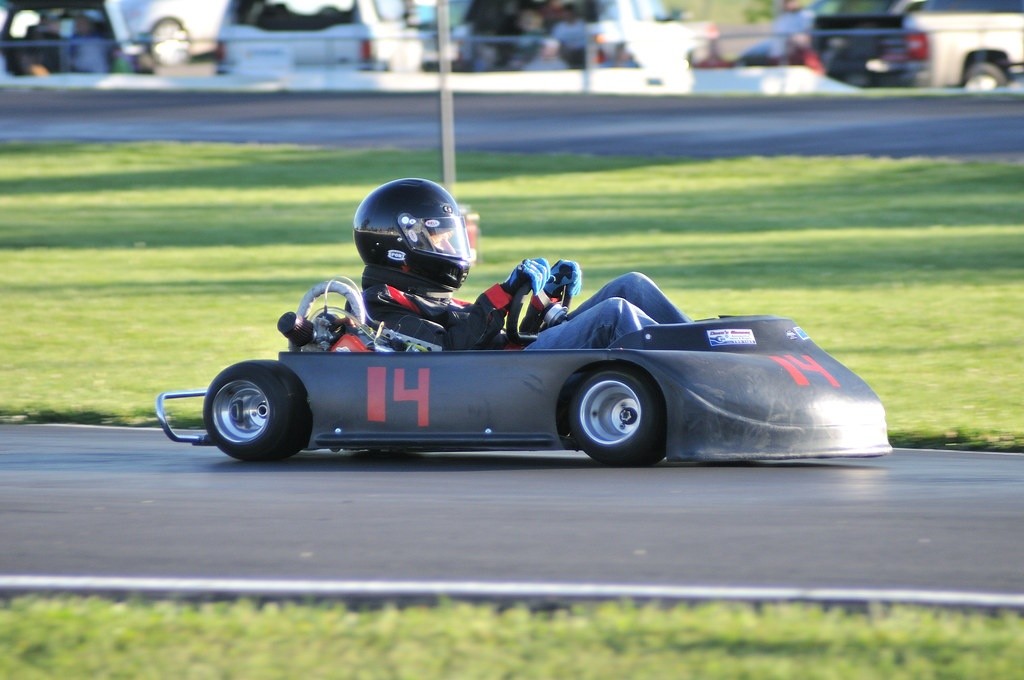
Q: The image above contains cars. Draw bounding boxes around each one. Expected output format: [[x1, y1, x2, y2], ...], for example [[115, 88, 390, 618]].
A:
[[690, 30, 858, 91], [216, 0, 425, 73], [422, 0, 706, 72], [0, 0, 226, 77]]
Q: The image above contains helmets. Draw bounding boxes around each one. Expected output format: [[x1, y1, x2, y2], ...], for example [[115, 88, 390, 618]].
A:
[[353, 178, 472, 290]]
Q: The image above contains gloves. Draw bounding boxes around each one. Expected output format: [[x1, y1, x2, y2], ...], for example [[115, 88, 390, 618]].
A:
[[504, 257, 554, 296], [544, 259, 582, 296]]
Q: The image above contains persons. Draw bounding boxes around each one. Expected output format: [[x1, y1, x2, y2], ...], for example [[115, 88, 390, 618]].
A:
[[350, 177, 695, 352], [434, 0, 650, 70], [786, 33, 827, 80], [765, 0, 818, 66]]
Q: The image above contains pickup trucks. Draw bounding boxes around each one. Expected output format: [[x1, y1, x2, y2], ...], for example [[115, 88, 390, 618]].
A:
[[811, 0, 1024, 90]]
